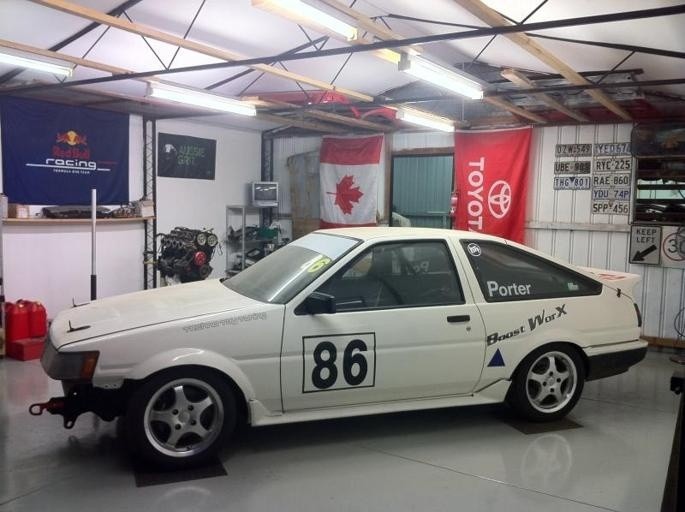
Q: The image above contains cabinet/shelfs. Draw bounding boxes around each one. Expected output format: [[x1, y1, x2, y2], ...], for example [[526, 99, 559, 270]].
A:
[[225, 204, 280, 281]]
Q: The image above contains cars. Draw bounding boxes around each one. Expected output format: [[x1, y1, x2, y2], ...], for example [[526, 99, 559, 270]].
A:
[[28, 225, 650, 469]]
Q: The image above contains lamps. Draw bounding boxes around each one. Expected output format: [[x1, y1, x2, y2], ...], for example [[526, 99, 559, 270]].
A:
[[395, 52, 484, 134], [253, 0, 357, 42], [147, 81, 257, 118]]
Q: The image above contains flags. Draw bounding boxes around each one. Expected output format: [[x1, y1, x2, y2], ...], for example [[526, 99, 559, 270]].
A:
[[319, 134, 384, 229], [451, 128, 532, 245]]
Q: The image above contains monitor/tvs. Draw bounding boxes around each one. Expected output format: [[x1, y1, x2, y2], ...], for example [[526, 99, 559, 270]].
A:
[[252, 182, 278, 206]]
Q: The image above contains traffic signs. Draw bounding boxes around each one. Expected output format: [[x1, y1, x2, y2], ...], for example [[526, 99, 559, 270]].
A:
[[629, 225, 664, 266], [661, 227, 685, 270]]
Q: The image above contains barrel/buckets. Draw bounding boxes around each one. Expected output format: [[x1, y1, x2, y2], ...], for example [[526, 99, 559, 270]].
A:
[[18, 298, 47, 337], [0, 301, 29, 340]]
